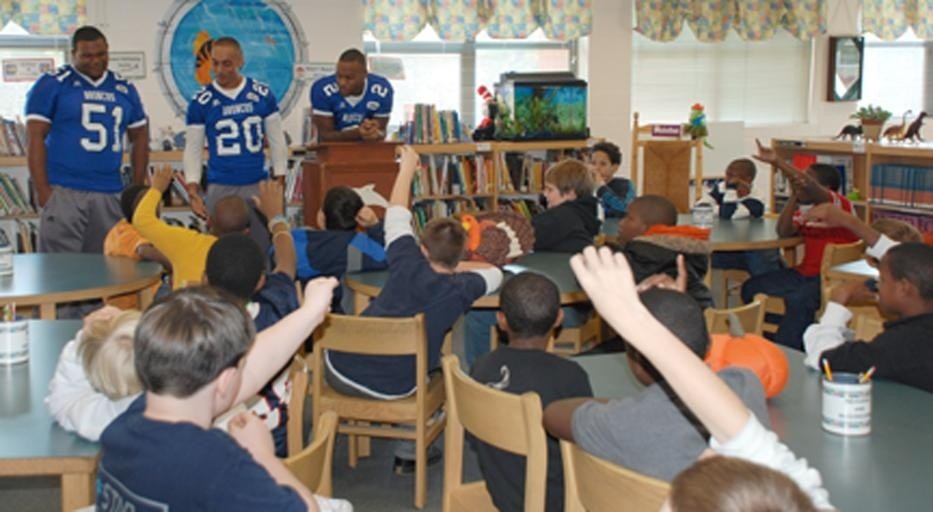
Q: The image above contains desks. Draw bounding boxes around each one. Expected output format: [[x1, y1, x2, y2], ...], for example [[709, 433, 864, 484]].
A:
[[569, 343, 933, 512], [0, 317, 103, 511], [0, 252, 163, 320]]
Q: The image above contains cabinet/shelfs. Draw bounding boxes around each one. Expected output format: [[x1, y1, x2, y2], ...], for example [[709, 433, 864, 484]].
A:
[[770, 140, 933, 243], [0, 137, 609, 254]]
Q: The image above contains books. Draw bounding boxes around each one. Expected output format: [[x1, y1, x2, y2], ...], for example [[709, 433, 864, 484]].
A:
[[774, 138, 933, 234], [0, 115, 38, 219], [397, 102, 595, 228]]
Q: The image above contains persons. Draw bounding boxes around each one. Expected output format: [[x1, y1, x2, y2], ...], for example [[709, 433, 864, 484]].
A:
[[310, 49, 393, 140], [584, 142, 636, 217], [468, 270, 593, 512], [184, 37, 287, 216], [462, 159, 603, 372], [96, 285, 320, 512], [690, 138, 933, 394], [322, 143, 504, 475], [542, 255, 771, 484], [45, 166, 385, 442], [569, 246, 839, 512], [25, 25, 150, 320]]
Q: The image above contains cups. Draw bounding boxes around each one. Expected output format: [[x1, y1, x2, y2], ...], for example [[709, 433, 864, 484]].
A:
[[0, 361, 31, 422], [692, 206, 714, 228], [1, 246, 13, 276], [0, 318, 29, 366], [821, 372, 872, 435]]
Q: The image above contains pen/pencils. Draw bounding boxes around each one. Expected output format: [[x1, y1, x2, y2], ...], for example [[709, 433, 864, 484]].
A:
[[823, 358, 832, 382], [859, 366, 877, 384]]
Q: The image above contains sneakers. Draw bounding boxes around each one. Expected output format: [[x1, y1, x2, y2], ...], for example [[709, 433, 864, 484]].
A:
[[393, 456, 442, 478]]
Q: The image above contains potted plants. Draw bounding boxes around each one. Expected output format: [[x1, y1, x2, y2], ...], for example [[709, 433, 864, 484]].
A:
[[850, 106, 893, 140]]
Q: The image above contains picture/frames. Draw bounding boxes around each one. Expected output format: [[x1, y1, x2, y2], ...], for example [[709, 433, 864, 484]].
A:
[[826, 35, 865, 102]]
